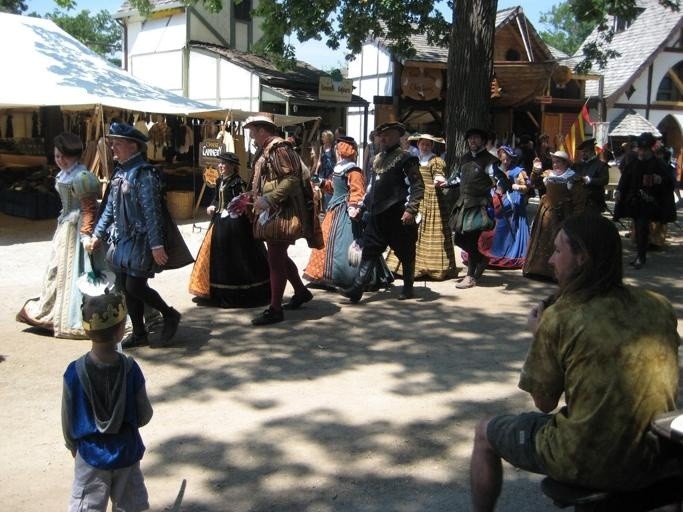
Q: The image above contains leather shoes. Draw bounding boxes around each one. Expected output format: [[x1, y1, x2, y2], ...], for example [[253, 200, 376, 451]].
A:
[[398, 286, 414, 299], [336, 283, 363, 303], [161, 307, 181, 338], [121, 332, 149, 347], [630, 256, 646, 269]]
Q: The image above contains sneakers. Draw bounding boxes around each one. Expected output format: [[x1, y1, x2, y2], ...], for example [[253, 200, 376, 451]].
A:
[[647, 243, 660, 251], [284, 288, 313, 309], [252, 306, 284, 326], [624, 232, 632, 238], [455, 274, 478, 288]]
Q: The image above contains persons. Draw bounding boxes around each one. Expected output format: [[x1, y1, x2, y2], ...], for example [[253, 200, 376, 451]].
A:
[[90, 122, 182, 350], [15, 133, 111, 341], [61, 291, 155, 512], [469, 211, 682, 511], [190, 113, 683, 326]]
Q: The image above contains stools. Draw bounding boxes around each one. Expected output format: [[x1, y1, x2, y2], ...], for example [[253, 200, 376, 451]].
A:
[[541, 442, 683, 512]]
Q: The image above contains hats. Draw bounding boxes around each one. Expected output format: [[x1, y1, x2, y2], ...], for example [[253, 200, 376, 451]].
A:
[[408, 133, 446, 144], [376, 122, 406, 137], [335, 137, 355, 144], [106, 123, 149, 143], [242, 115, 278, 131], [464, 128, 488, 145], [576, 138, 596, 150], [636, 132, 655, 149], [54, 132, 83, 157], [216, 152, 241, 165], [497, 145, 517, 157], [550, 150, 570, 161]]
